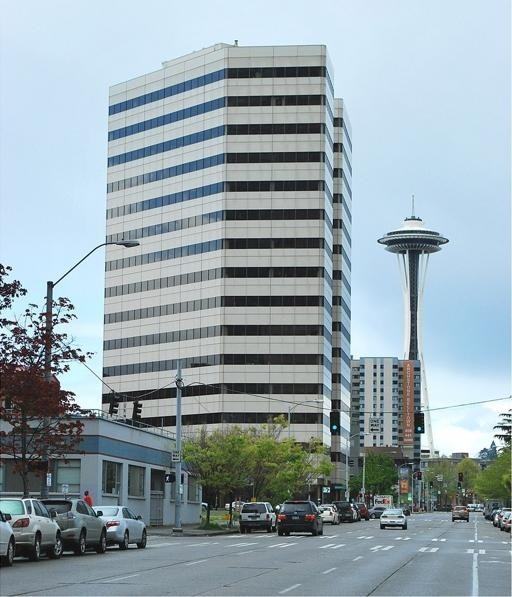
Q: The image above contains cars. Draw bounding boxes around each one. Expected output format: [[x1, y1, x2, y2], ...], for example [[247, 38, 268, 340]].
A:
[[451, 498, 512, 532], [202, 496, 411, 535], [0, 492, 151, 567]]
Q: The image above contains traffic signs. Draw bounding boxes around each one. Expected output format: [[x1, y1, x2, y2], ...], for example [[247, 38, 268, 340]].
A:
[[172, 449, 182, 463], [369, 416, 381, 433]]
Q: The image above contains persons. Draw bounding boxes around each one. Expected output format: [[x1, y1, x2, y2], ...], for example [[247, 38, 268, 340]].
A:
[[83, 489, 93, 507]]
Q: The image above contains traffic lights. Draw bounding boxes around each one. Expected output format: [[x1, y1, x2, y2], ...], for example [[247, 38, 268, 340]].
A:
[[164, 473, 175, 482], [413, 412, 425, 434], [417, 470, 423, 480], [458, 482, 462, 489], [108, 392, 121, 414], [429, 481, 433, 489], [132, 398, 142, 420], [458, 472, 464, 481], [329, 411, 340, 435]]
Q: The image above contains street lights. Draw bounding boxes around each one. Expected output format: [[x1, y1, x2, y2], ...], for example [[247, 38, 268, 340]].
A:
[[382, 450, 399, 455], [286, 396, 326, 439], [38, 236, 143, 498], [397, 462, 415, 503], [345, 432, 372, 500]]
